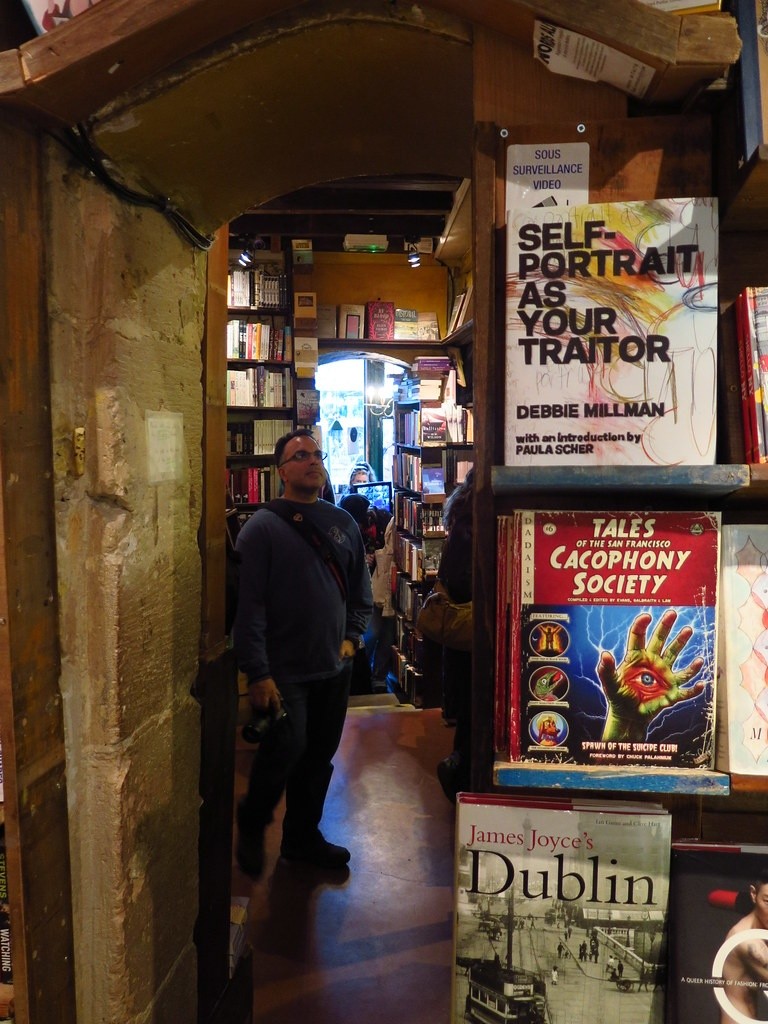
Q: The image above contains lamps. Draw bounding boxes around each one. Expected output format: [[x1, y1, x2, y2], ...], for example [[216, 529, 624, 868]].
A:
[[238, 241, 256, 269], [406, 243, 421, 268]]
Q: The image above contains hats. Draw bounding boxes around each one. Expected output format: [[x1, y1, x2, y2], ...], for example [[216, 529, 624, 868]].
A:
[[337, 494, 370, 518]]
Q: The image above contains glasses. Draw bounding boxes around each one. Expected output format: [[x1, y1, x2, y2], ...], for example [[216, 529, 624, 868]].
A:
[[279, 451, 328, 467]]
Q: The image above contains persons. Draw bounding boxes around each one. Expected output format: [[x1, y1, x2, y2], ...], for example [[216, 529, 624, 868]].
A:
[[340, 461, 376, 497], [228, 427, 376, 882], [718, 870, 768, 1023], [434, 463, 476, 803], [487, 908, 651, 996], [338, 495, 399, 695]]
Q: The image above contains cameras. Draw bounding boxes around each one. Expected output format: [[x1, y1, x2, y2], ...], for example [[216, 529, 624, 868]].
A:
[[242, 696, 288, 744]]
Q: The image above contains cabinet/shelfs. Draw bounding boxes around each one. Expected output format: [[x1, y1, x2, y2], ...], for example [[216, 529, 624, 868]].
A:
[[226, 116, 768, 844]]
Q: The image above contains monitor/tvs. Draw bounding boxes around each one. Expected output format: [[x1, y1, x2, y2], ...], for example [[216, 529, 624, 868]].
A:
[[354, 482, 393, 515]]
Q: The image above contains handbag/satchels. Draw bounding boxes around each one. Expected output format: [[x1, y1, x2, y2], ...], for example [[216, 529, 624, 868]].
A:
[[416, 581, 473, 651]]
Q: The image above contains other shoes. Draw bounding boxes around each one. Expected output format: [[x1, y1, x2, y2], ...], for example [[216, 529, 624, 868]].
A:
[[437, 760, 471, 806], [371, 678, 387, 694], [280, 831, 350, 866], [236, 799, 266, 880]]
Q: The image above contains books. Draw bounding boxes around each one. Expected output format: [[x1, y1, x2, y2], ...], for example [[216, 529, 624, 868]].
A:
[[319, 300, 337, 339], [337, 302, 367, 339], [393, 347, 475, 702], [452, 198, 768, 1023], [364, 300, 396, 342], [417, 309, 441, 342], [224, 233, 319, 514], [392, 306, 420, 341], [443, 286, 477, 336]]
